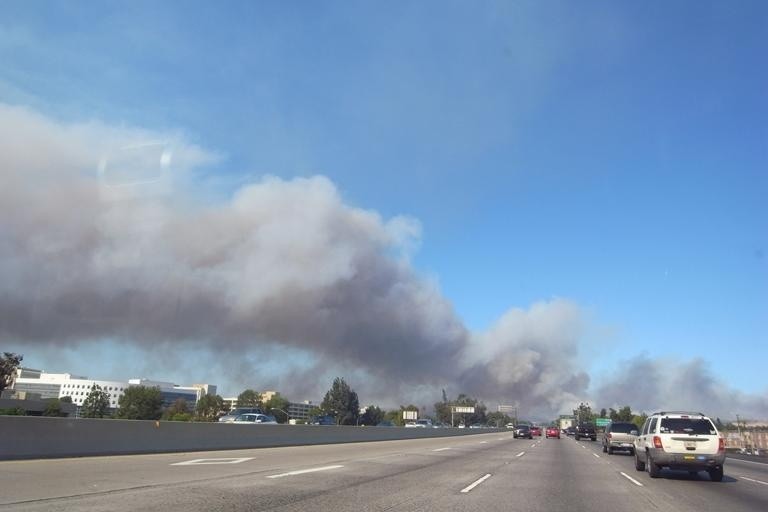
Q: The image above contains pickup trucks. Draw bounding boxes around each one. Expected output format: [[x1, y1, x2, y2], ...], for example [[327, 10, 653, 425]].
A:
[[603, 423, 639, 454]]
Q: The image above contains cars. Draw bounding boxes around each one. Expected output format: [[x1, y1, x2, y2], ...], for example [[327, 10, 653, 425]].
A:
[[219, 408, 276, 424], [305, 417, 336, 425], [513, 425, 596, 441], [405, 419, 450, 428]]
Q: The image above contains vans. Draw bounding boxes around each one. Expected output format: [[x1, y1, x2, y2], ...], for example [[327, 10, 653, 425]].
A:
[[633, 410, 725, 481]]
